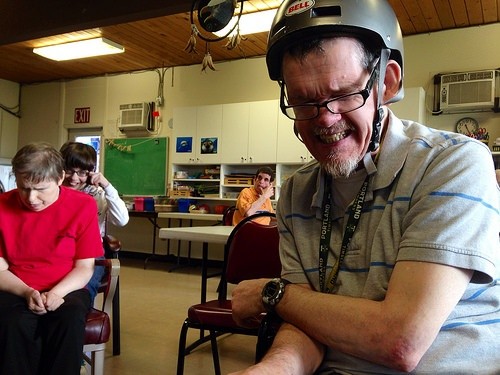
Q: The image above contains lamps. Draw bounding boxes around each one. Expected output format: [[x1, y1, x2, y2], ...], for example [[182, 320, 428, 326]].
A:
[[211, 1, 280, 38], [32, 37, 125, 62]]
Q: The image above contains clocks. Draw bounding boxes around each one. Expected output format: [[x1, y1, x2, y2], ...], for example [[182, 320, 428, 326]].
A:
[[456, 117, 479, 135]]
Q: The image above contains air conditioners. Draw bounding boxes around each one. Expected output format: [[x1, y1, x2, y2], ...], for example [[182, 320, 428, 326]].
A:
[[119, 102, 150, 131], [440, 70, 494, 114]]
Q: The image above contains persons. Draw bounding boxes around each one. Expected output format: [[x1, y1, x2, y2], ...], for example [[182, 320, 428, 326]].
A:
[[229, 0, 500, 375], [233, 167, 276, 226], [0, 142, 129, 375]]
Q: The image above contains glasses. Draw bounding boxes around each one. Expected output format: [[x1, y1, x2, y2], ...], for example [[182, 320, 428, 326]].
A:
[[65, 169, 88, 177], [280, 56, 381, 120]]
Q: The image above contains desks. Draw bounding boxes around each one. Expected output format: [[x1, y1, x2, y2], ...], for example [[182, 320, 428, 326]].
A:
[[158, 226, 237, 303]]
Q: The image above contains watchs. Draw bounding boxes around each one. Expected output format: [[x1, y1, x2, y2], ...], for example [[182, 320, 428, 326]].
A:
[[261, 277, 289, 322]]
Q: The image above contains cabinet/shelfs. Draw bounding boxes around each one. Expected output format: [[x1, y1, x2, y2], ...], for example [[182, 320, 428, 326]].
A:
[[169, 98, 316, 200]]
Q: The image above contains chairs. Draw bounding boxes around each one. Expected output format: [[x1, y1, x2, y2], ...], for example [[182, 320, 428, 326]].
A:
[[78, 205, 282, 375]]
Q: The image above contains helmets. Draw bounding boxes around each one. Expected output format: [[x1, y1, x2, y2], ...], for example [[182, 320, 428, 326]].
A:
[[266, 0, 405, 81]]
